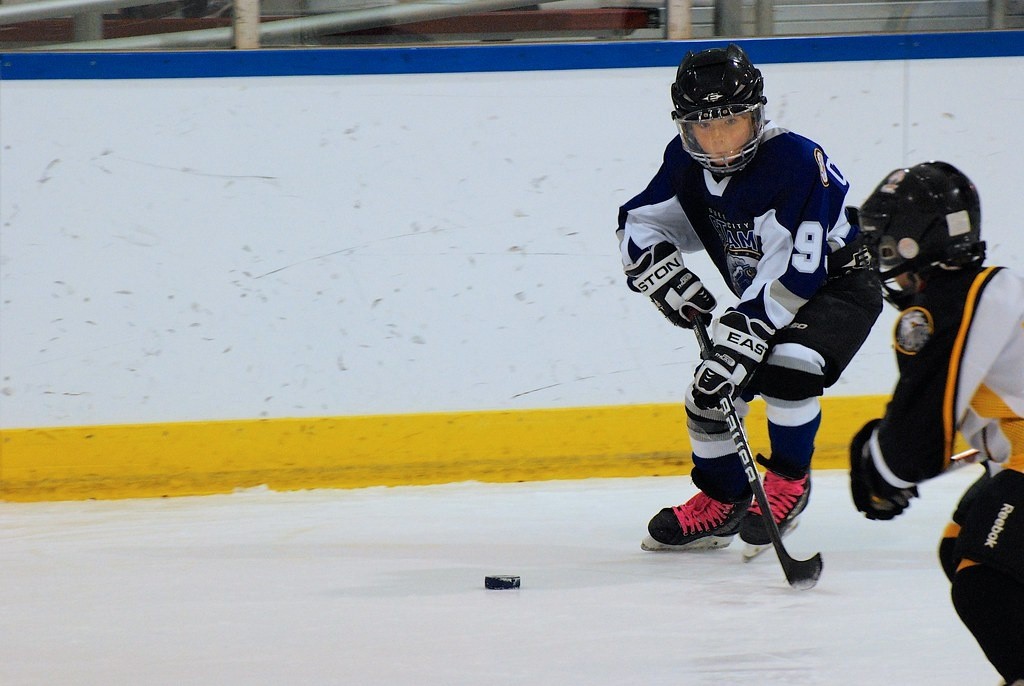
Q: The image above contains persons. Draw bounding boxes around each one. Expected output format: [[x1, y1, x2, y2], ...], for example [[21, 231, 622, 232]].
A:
[[615, 45, 884, 562], [845, 160, 1024, 686]]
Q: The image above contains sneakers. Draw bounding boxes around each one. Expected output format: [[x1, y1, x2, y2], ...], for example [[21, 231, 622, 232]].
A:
[[740, 453, 811, 562], [641, 466, 753, 552]]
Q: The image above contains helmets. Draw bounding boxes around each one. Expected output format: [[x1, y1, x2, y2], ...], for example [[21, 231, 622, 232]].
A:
[[670, 44, 769, 173], [856, 161, 987, 312]]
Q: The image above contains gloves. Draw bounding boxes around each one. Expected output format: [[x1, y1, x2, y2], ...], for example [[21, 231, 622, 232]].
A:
[[691, 307, 775, 414], [625, 240, 716, 330], [851, 431, 918, 520]]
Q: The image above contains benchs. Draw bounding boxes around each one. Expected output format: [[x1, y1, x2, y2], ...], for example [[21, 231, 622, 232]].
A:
[[0, 4, 656, 45]]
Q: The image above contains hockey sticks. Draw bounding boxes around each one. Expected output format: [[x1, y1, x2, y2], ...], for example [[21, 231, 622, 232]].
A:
[[688, 305, 824, 592]]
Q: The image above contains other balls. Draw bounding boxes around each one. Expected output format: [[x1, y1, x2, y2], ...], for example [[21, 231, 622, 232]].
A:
[[484, 574, 521, 591]]
